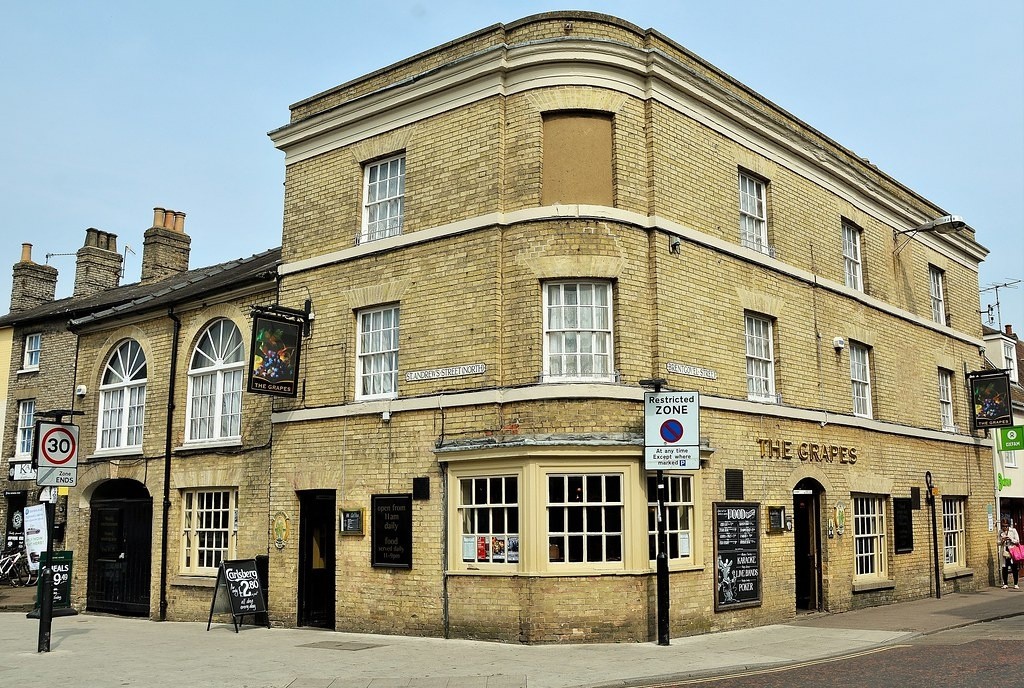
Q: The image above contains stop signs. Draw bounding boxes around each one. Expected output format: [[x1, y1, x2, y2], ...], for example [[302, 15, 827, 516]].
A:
[[658, 418, 684, 444]]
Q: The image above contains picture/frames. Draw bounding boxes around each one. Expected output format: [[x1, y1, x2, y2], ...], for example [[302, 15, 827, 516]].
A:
[[971, 374, 1014, 430], [246, 313, 303, 398]]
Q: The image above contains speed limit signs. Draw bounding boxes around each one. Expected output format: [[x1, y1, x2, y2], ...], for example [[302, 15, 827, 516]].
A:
[[36, 424, 80, 487]]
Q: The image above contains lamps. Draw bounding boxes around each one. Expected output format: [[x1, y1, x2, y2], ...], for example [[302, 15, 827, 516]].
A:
[[893, 215, 965, 241]]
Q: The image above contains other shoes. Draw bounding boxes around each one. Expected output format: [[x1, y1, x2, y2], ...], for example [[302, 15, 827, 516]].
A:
[[1001, 585, 1008, 588], [1014, 585, 1019, 588]]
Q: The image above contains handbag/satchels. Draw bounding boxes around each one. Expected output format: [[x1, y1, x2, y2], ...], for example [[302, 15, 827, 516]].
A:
[[1008, 540, 1024, 562]]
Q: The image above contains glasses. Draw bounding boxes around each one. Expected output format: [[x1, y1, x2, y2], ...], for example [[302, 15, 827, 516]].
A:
[[1002, 525, 1009, 527]]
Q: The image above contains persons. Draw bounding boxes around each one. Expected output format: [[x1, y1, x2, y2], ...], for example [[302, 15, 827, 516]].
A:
[[997, 518, 1021, 589]]
